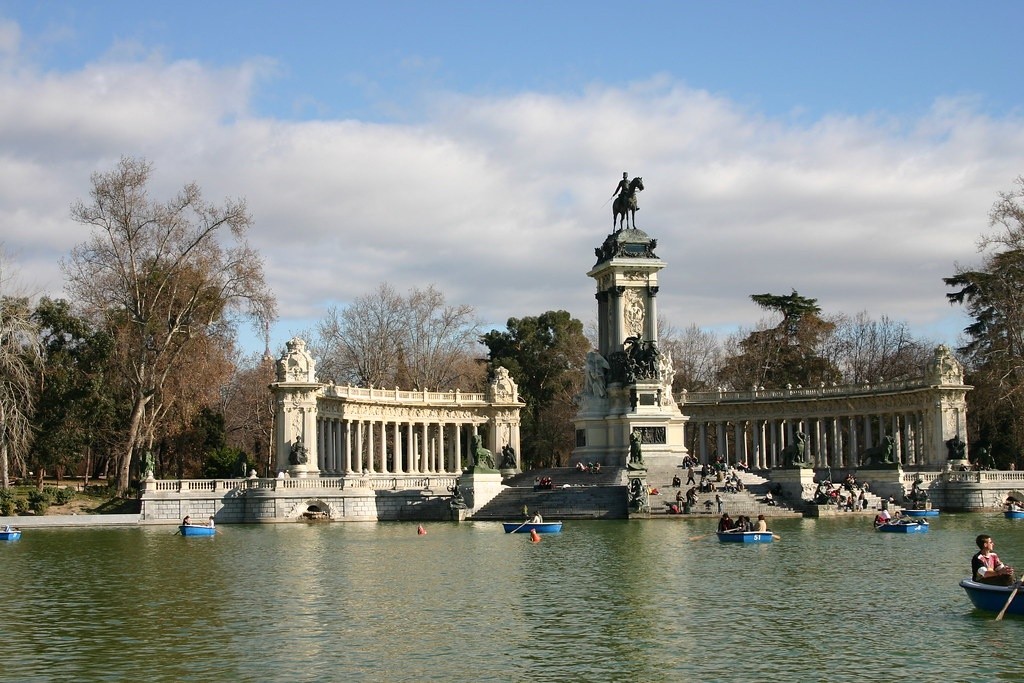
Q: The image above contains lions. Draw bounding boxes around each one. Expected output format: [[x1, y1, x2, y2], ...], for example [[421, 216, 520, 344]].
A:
[[470, 435, 495, 469], [630, 431, 642, 463], [858, 435, 896, 466], [780, 430, 806, 464]]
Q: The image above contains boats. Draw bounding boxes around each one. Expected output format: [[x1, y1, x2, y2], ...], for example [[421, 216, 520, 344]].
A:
[[0, 530, 22, 543], [1002, 509, 1024, 519], [876, 520, 930, 535], [501, 521, 562, 535], [177, 524, 217, 537], [716, 528, 774, 544], [957, 578, 1024, 618], [898, 508, 941, 518]]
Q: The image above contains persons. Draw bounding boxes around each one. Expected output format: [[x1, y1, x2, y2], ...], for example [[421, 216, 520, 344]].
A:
[[587, 365, 606, 397], [554, 450, 560, 467], [203, 516, 214, 526], [672, 453, 748, 514], [530, 528, 541, 543], [575, 460, 600, 474], [531, 511, 543, 523], [629, 432, 642, 464], [14, 528, 20, 531], [624, 332, 657, 353], [765, 482, 782, 505], [646, 485, 663, 496], [588, 351, 603, 372], [958, 462, 1016, 471], [288, 436, 307, 462], [183, 516, 192, 525], [418, 524, 427, 535], [722, 512, 766, 531], [502, 444, 516, 465], [629, 356, 653, 380], [612, 172, 640, 211], [533, 476, 552, 491], [631, 478, 643, 502], [522, 504, 528, 514], [828, 474, 1024, 585], [447, 484, 465, 510]]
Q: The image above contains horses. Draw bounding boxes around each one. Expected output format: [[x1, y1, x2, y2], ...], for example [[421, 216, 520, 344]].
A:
[[613, 177, 644, 233]]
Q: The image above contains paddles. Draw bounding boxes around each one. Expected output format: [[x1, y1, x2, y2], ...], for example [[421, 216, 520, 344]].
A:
[[994, 575, 1024, 621], [906, 515, 911, 519], [872, 514, 907, 530], [689, 527, 740, 541], [200, 519, 223, 534], [174, 529, 181, 535], [510, 519, 531, 534]]
